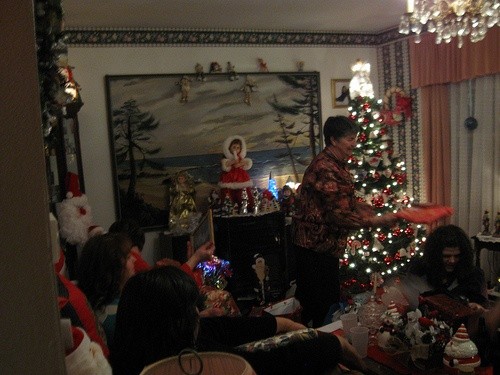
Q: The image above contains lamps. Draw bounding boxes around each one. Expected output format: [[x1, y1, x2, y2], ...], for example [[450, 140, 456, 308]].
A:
[[286, 176, 294, 189], [399, 0, 500, 49]]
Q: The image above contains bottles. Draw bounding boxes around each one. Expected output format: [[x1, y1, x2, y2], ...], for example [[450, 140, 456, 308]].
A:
[[480, 210, 492, 237], [492, 213, 500, 238]]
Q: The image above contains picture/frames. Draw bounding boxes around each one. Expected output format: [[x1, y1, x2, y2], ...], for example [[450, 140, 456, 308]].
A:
[[105, 71, 324, 235], [191, 209, 215, 257], [331, 79, 349, 108]]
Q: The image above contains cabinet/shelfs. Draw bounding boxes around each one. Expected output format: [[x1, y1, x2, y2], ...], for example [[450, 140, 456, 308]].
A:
[[171, 214, 293, 296]]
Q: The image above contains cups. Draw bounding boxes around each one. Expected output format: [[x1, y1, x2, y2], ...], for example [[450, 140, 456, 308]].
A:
[[351, 326, 369, 356], [341, 314, 357, 332]]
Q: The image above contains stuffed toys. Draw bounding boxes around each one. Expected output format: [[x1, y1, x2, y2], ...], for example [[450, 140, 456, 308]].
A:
[[56, 172, 92, 245]]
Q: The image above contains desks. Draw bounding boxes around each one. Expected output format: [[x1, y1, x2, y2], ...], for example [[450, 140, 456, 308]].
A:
[[473, 235, 500, 301]]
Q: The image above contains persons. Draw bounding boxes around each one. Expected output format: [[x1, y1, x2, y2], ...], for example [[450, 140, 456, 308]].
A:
[[219, 136, 254, 208], [110, 216, 215, 286], [79, 235, 224, 357], [109, 265, 361, 375], [165, 166, 200, 233], [408, 224, 489, 316], [281, 116, 397, 329]]
